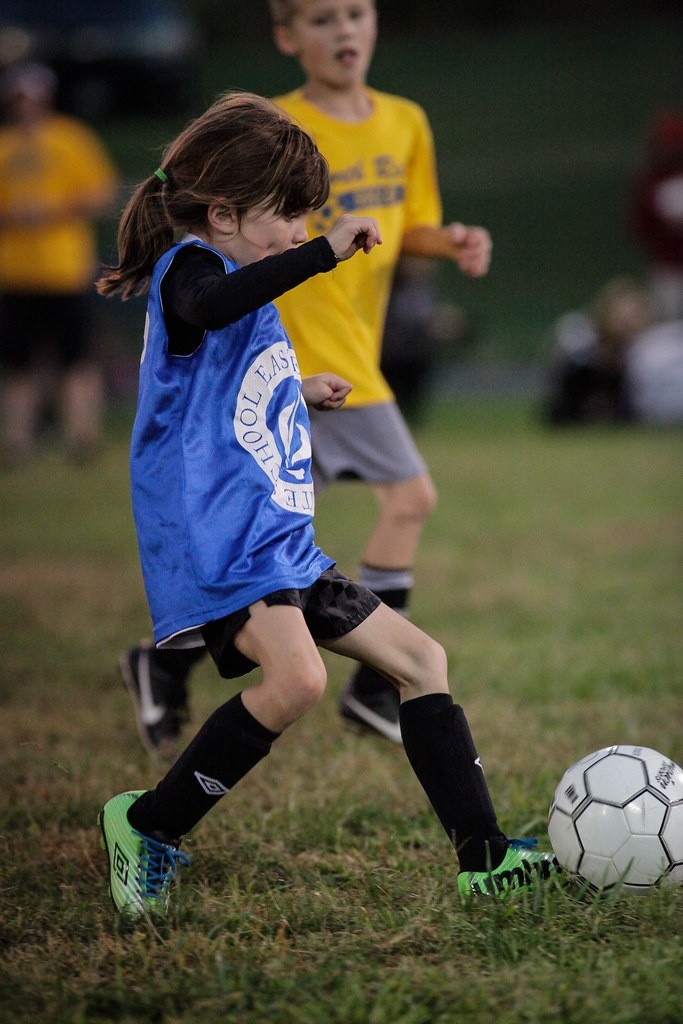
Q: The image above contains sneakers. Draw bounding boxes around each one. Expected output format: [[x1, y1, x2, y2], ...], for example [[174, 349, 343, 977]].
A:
[[96, 790, 189, 925], [115, 638, 193, 766], [458, 837, 562, 907], [336, 673, 403, 746]]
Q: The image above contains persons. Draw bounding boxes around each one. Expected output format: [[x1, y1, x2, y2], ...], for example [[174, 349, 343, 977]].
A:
[[119, 0, 494, 773], [542, 277, 647, 433], [93, 94, 560, 931], [0, 62, 118, 471]]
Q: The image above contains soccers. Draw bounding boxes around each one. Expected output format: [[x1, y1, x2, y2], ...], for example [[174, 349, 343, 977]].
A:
[[548, 745, 683, 905]]
